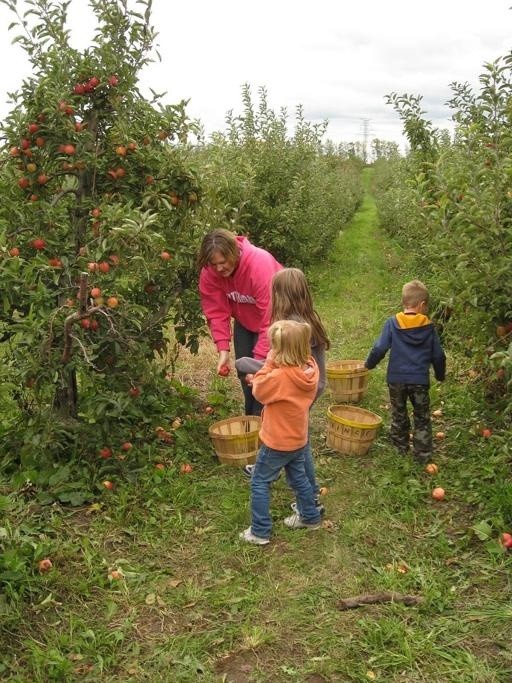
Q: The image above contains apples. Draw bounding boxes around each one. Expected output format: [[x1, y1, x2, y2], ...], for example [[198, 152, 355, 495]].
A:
[[39, 559, 53, 571], [485, 320, 512, 381], [246, 373, 256, 384], [482, 429, 491, 438], [432, 487, 445, 501], [435, 432, 445, 440], [218, 366, 230, 378], [99, 386, 216, 491], [498, 533, 512, 549], [7, 76, 198, 332], [427, 463, 438, 475], [111, 571, 123, 581], [433, 409, 443, 418]]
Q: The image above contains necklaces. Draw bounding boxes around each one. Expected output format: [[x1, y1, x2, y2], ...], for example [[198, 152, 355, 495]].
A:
[[403, 311, 416, 315]]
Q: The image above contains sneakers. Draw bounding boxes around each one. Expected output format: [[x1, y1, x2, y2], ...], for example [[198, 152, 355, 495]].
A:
[[290, 499, 324, 515], [239, 525, 270, 544], [243, 465, 281, 480], [284, 503, 319, 528]]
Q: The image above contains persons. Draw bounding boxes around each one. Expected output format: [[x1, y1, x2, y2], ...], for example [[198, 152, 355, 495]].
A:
[[360, 278, 449, 461], [196, 226, 284, 417], [233, 321, 322, 546], [233, 266, 330, 517]]
